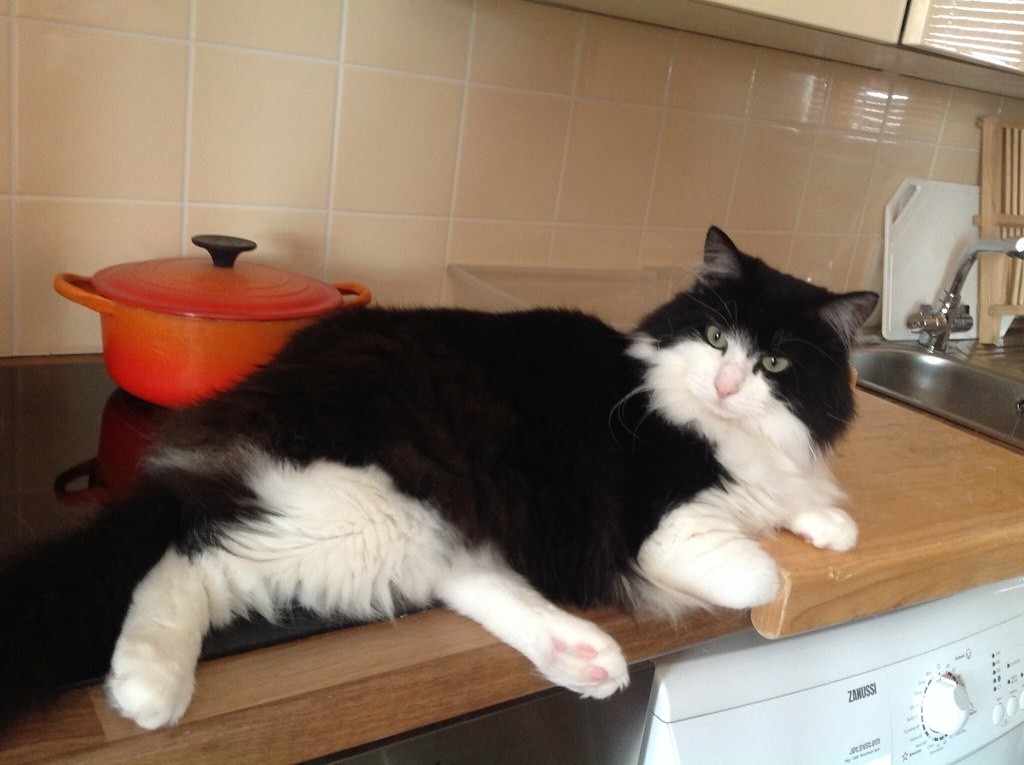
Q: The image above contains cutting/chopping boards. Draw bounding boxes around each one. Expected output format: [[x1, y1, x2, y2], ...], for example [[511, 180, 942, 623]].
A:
[[881, 178, 1016, 341]]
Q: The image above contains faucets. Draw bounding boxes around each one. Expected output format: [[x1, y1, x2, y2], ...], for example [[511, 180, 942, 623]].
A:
[[907, 236, 1024, 353]]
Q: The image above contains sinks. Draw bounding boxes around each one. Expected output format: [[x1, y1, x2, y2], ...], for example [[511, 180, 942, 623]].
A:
[[847, 339, 1024, 451]]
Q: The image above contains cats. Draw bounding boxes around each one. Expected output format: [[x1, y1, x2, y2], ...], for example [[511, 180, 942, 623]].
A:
[[0, 225, 882, 731]]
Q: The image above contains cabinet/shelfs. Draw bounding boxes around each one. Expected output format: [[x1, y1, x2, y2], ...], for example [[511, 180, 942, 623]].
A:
[[533, 0, 1024, 102]]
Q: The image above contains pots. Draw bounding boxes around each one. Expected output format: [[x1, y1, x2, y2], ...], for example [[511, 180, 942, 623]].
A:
[[53, 235, 371, 408], [54, 388, 176, 508]]
